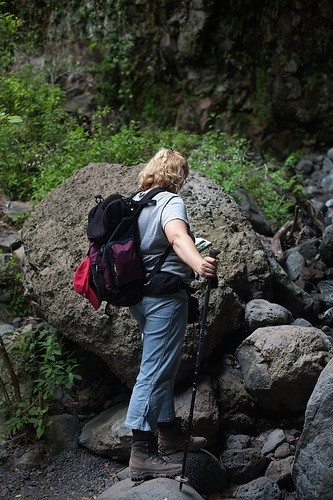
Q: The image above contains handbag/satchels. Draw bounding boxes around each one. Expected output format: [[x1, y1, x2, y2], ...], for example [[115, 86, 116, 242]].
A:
[[144, 268, 192, 297]]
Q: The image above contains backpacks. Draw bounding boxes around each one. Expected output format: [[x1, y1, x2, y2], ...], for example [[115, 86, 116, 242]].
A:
[[87, 186, 178, 307]]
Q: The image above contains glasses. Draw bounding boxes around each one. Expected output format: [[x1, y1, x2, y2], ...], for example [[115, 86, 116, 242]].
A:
[[182, 178, 187, 185]]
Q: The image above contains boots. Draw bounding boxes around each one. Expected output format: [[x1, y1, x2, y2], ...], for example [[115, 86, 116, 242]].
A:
[[157, 424, 207, 456], [127, 429, 183, 479]]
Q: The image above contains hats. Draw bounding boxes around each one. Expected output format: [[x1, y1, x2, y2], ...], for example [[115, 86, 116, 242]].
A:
[[73, 255, 102, 311]]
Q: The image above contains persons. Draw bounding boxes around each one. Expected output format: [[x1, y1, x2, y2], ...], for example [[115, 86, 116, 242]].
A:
[[124, 147, 221, 480]]
